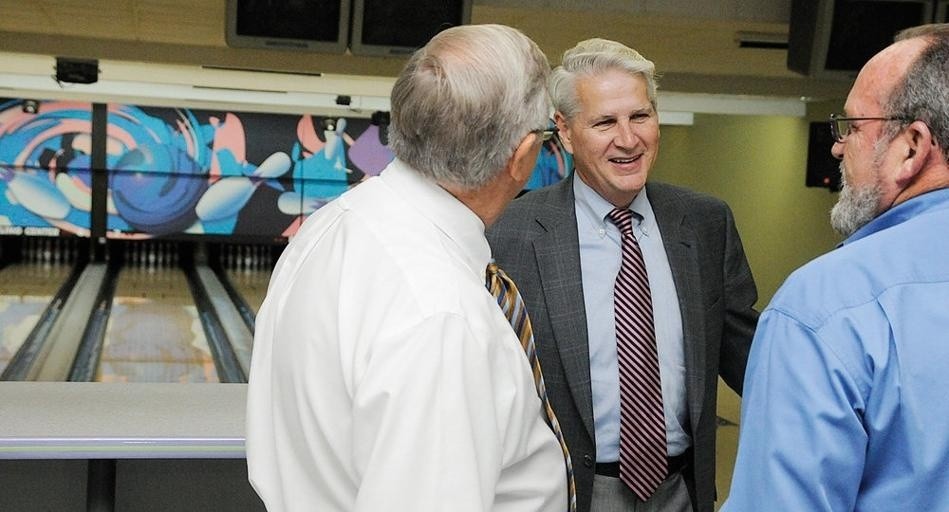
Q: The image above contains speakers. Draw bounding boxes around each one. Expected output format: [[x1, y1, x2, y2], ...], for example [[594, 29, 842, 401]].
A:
[[56, 56, 98, 84], [336, 96, 351, 105], [805, 124, 843, 190]]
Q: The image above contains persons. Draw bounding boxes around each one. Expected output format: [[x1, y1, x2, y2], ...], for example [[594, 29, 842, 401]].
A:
[[470, 34, 768, 512], [243, 23, 581, 510], [708, 20, 948, 512]]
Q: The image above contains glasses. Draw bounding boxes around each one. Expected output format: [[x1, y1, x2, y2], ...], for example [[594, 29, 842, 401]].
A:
[[830, 109, 915, 145]]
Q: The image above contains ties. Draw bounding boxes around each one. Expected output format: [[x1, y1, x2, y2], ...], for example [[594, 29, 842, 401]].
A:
[[605, 207, 670, 503], [485, 260, 577, 511]]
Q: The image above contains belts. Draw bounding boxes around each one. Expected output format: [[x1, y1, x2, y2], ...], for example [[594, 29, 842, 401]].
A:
[[595, 455, 688, 479]]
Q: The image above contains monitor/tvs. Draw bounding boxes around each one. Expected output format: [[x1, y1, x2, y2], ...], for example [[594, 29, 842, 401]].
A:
[[787, 0, 934, 82], [225, 0, 350, 54], [350, 1, 473, 58]]
